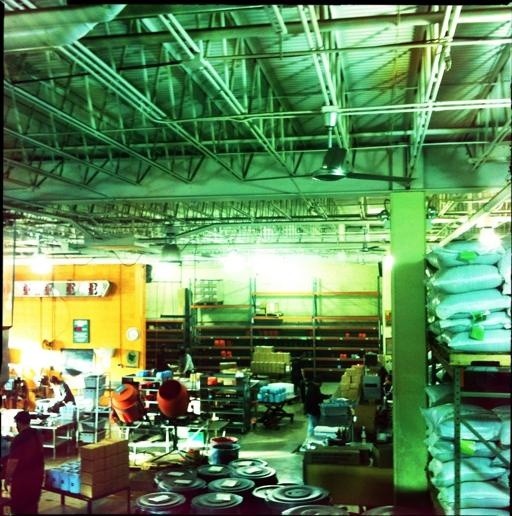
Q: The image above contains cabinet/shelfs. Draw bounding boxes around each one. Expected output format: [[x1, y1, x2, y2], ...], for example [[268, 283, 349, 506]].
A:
[[74, 362, 113, 447], [426, 328, 512, 516], [145, 278, 384, 432]]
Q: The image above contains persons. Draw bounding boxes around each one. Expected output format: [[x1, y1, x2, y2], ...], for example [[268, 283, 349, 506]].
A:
[[297, 377, 333, 450], [0, 409, 45, 515], [289, 353, 308, 402], [176, 346, 195, 377], [382, 374, 394, 400], [49, 376, 76, 407]]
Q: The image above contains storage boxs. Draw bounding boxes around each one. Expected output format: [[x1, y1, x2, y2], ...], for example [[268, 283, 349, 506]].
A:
[[78, 437, 131, 497]]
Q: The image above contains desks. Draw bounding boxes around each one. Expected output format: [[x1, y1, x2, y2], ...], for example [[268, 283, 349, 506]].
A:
[[37, 459, 134, 512]]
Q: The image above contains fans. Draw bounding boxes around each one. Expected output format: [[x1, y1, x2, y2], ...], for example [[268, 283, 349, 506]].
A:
[[240, 110, 418, 183]]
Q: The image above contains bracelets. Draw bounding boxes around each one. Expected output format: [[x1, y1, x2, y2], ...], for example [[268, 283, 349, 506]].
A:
[[383, 386, 386, 388]]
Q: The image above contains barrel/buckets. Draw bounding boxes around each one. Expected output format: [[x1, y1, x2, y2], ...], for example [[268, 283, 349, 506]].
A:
[[135, 436, 422, 515], [111, 383, 145, 424], [156, 379, 190, 419]]
